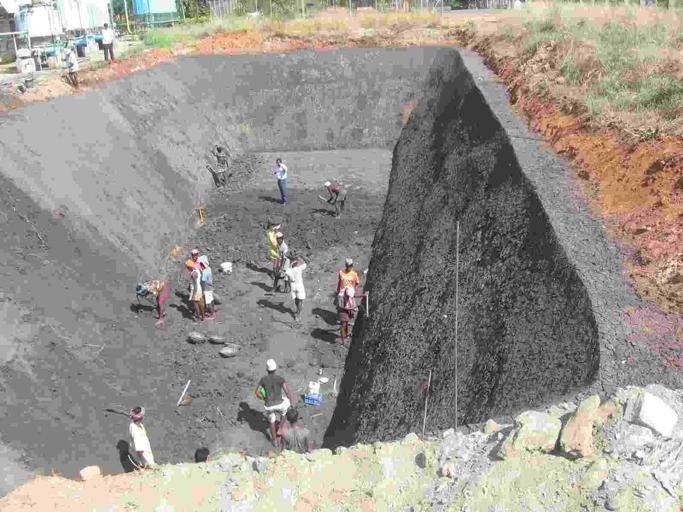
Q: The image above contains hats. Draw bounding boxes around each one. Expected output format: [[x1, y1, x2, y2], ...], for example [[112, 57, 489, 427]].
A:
[[185, 249, 209, 268], [136, 286, 148, 296], [346, 287, 355, 297], [266, 359, 276, 371], [345, 258, 353, 267], [276, 232, 284, 238], [324, 181, 331, 186]]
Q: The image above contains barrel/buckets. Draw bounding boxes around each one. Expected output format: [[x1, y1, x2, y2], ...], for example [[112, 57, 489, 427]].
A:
[[79, 464, 101, 479], [309, 381, 320, 395], [220, 261, 233, 272]]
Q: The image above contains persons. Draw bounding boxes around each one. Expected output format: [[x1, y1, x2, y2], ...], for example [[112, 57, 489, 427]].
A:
[[184, 248, 216, 321], [211, 144, 229, 186], [136, 279, 169, 326], [63, 48, 81, 90], [195, 447, 210, 463], [267, 222, 307, 322], [324, 181, 347, 220], [255, 358, 312, 453], [127, 406, 155, 467], [336, 256, 360, 345], [101, 23, 115, 64], [274, 157, 288, 206]]
